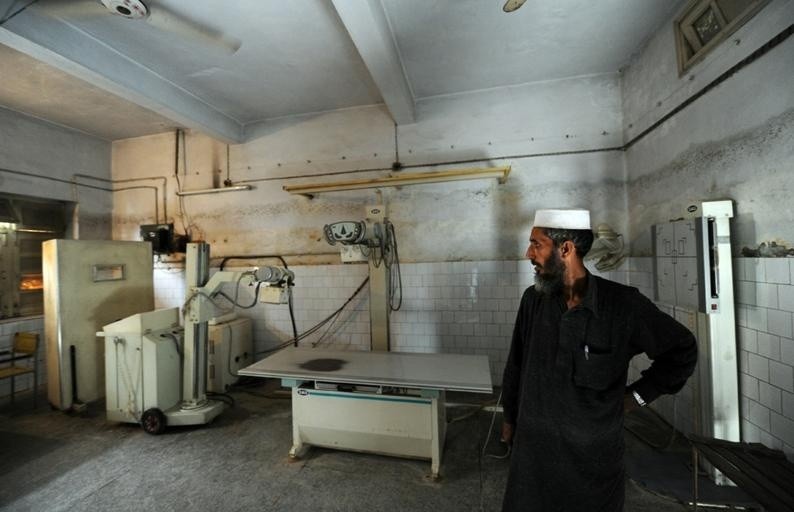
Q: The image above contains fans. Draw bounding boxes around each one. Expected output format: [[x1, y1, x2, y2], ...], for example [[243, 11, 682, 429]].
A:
[[21, 1, 243, 55]]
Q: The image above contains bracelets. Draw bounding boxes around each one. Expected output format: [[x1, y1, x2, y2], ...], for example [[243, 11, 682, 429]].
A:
[[632, 390, 647, 408]]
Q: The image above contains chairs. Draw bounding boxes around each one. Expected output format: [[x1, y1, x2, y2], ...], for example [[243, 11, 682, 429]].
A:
[[0, 332, 40, 419]]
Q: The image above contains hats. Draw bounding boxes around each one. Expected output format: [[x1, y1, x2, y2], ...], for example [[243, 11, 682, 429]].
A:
[[533, 209, 590, 230]]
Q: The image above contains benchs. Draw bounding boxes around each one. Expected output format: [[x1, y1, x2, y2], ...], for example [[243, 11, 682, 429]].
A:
[[685, 434, 794, 512]]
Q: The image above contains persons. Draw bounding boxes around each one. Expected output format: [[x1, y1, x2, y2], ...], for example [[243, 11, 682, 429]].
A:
[[502, 208, 697, 512]]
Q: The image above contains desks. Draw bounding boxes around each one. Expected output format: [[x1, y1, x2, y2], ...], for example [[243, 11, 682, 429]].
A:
[[236, 346, 494, 482]]
